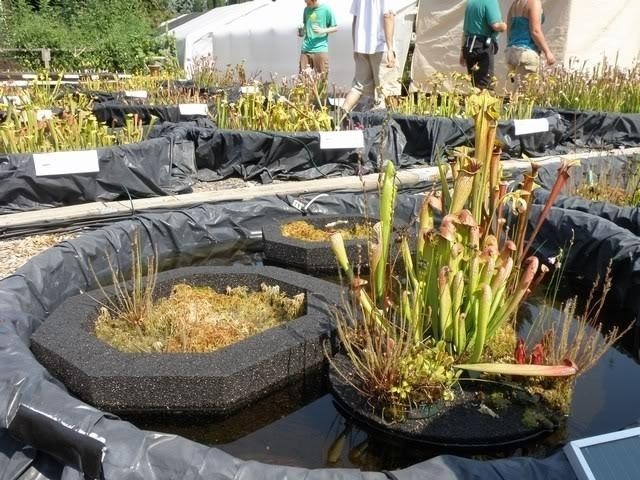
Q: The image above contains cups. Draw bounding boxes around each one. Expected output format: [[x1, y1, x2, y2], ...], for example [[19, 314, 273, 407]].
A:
[[311, 22, 319, 35], [298, 24, 305, 38]]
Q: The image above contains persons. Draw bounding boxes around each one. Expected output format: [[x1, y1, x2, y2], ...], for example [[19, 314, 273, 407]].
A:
[[342, 0, 396, 112], [297, 0, 340, 80], [460, 0, 507, 90], [507, 0, 556, 75]]
